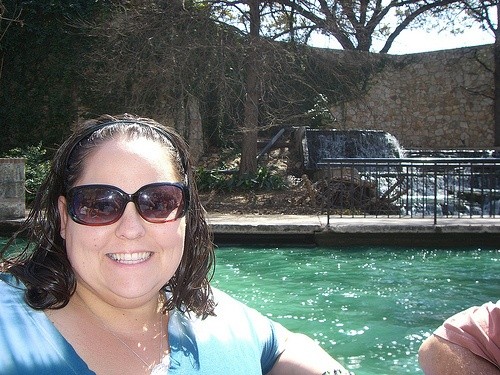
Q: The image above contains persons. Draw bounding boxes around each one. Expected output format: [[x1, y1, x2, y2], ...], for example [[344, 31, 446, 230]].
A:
[[0, 111, 353, 375], [417, 299, 500, 375]]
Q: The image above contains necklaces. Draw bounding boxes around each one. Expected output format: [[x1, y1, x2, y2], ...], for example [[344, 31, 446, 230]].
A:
[[102, 309, 163, 375]]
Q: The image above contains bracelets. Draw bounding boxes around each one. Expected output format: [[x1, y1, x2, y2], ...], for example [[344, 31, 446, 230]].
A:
[[321, 369, 352, 375]]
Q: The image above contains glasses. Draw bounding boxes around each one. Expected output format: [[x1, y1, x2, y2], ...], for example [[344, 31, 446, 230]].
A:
[[62, 183, 190, 226]]
